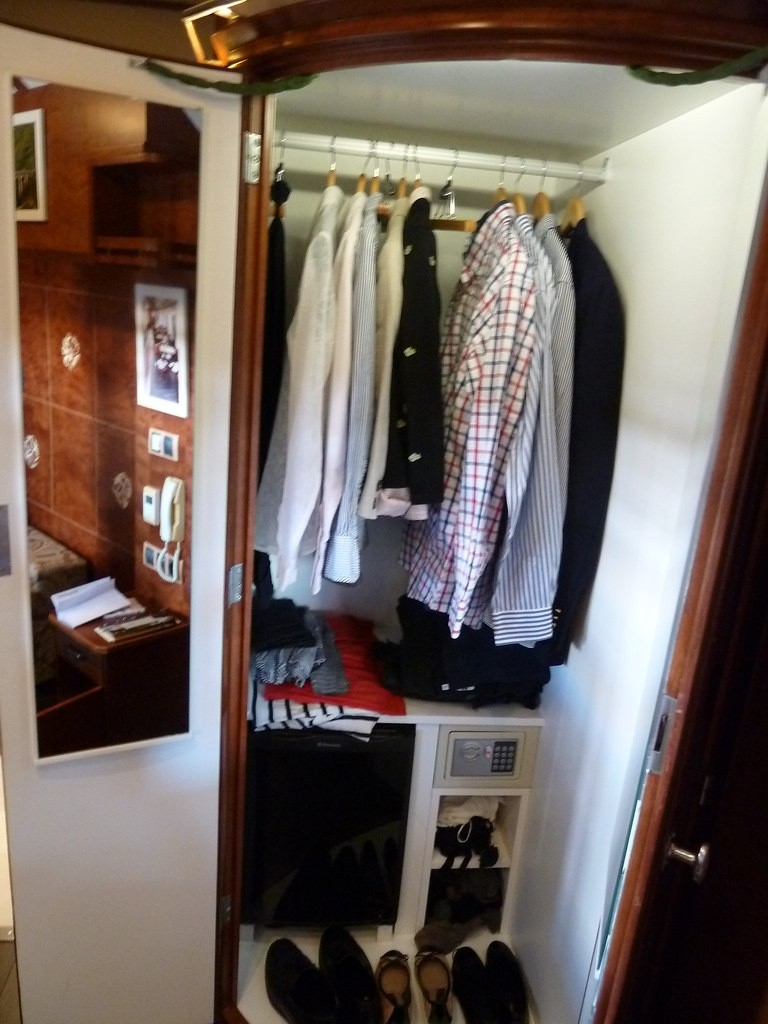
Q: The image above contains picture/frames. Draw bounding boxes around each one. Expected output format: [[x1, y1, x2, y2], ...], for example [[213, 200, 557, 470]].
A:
[[12, 106, 47, 222], [134, 280, 189, 418]]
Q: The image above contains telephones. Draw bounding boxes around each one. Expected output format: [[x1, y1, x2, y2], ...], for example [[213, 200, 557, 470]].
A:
[[159, 476, 185, 543]]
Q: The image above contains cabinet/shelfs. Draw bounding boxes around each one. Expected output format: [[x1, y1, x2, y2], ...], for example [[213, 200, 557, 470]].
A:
[[44, 594, 189, 744], [177, 1, 768, 1023]]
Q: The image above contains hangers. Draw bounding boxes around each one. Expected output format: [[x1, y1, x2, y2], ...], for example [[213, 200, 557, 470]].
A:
[[270, 126, 586, 231]]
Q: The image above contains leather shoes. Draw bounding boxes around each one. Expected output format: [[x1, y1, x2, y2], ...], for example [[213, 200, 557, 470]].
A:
[[452, 940, 529, 1024], [319, 926, 386, 1024], [265, 938, 345, 1024]]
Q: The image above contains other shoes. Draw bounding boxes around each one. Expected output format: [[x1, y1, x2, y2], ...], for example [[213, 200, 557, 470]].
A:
[[414, 950, 454, 1024], [375, 950, 413, 1024]]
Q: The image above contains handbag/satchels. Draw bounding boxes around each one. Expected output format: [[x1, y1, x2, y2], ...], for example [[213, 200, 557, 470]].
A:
[[394, 593, 559, 711]]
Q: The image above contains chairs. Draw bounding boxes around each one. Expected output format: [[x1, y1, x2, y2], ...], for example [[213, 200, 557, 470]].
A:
[[25, 522, 87, 684]]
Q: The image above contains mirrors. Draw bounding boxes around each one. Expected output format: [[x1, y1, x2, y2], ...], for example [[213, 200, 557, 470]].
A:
[[9, 74, 207, 765]]
